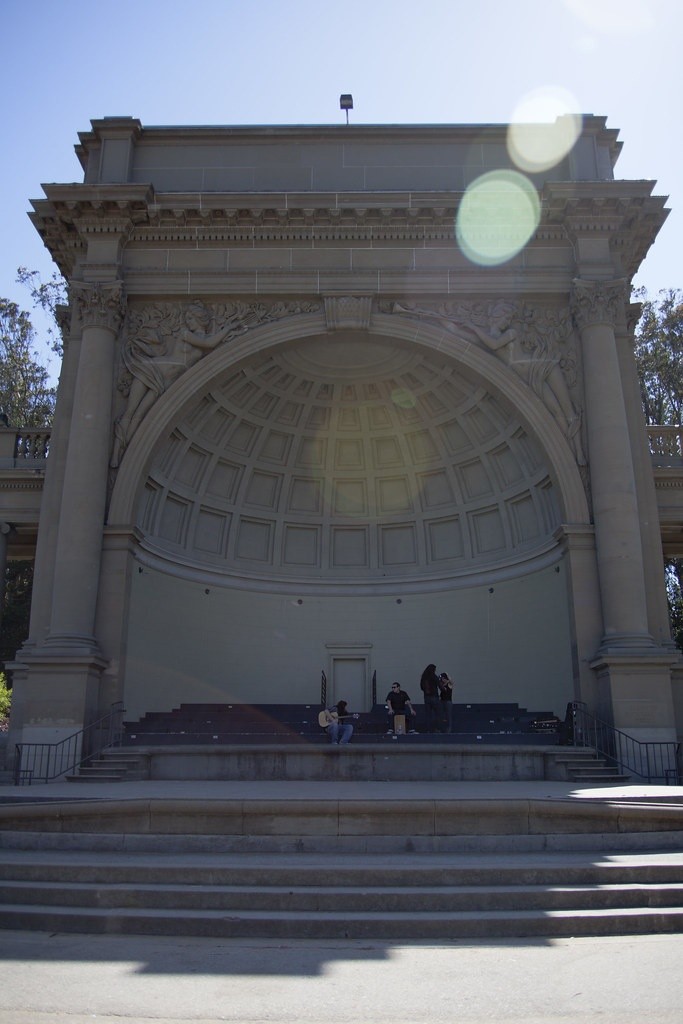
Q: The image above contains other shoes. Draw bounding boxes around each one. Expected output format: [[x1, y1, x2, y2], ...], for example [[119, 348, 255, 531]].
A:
[[387, 728, 393, 735], [408, 730, 420, 735], [339, 740, 352, 744], [331, 741, 338, 745]]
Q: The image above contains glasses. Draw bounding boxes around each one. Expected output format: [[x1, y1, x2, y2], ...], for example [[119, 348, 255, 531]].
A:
[[392, 686, 398, 689]]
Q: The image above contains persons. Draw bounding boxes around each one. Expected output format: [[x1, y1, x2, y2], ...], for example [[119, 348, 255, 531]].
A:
[[419, 663, 449, 733], [438, 673, 453, 733], [323, 700, 353, 745], [439, 311, 587, 466], [385, 682, 418, 734], [109, 299, 250, 469]]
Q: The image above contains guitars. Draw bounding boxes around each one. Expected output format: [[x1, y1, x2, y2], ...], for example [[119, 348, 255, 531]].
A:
[[318, 710, 360, 728]]
